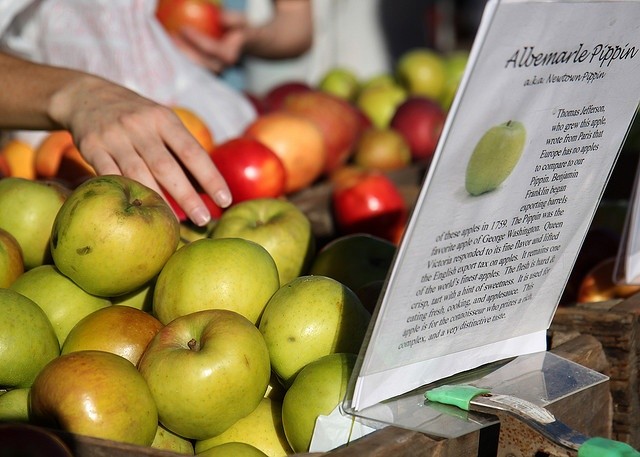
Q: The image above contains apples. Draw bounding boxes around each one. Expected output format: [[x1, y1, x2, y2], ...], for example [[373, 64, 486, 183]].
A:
[[0, 230, 25, 292], [134, 310, 271, 440], [245, 113, 324, 193], [153, 237, 280, 325], [210, 199, 313, 285], [61, 305, 164, 367], [0, 288, 61, 388], [3, 181, 72, 268], [320, 72, 358, 102], [465, 121, 525, 196], [193, 400, 288, 456], [333, 173, 407, 228], [50, 174, 179, 299], [155, 2, 222, 45], [360, 50, 470, 173], [152, 425, 192, 453], [313, 234, 397, 290], [26, 350, 159, 447], [1, 387, 29, 420], [260, 275, 371, 384], [195, 442, 269, 457], [112, 288, 152, 312], [0, 132, 97, 190], [171, 105, 213, 154], [282, 354, 355, 452], [286, 93, 360, 173], [9, 265, 112, 347], [211, 137, 286, 200], [0, 422, 69, 453]]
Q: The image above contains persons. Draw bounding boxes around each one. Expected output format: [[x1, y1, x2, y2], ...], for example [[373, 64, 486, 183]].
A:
[[0, 1, 256, 232], [170, 0, 389, 93]]
